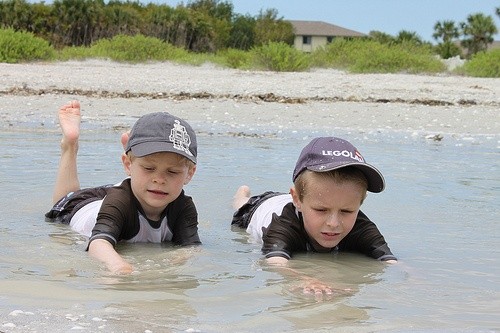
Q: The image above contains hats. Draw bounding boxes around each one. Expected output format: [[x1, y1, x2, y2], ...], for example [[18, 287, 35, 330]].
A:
[[292, 138, 386, 193], [125, 112, 198, 165]]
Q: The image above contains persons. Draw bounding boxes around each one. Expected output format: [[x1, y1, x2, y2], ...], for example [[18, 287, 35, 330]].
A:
[[45, 101, 202, 264], [231, 137, 397, 303]]
